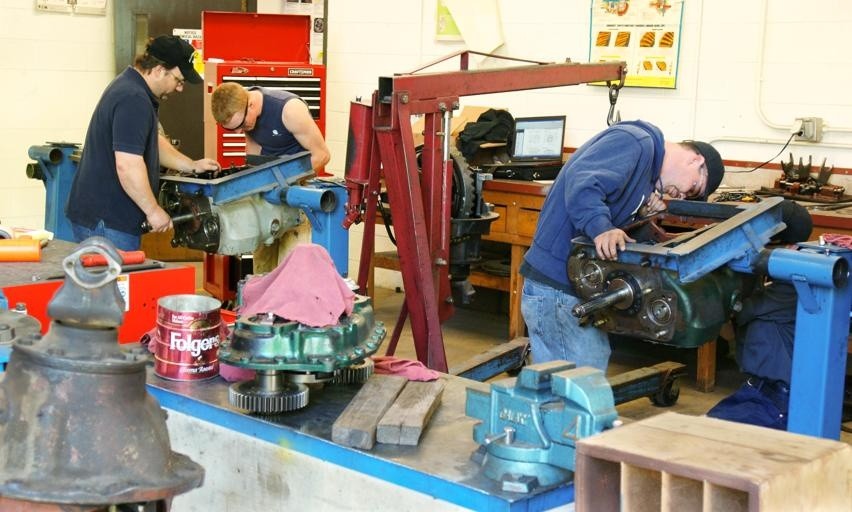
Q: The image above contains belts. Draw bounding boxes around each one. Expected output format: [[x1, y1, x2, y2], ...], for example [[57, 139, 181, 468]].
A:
[[746, 376, 790, 416]]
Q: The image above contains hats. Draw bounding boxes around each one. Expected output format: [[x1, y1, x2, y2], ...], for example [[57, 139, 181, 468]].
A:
[[686, 140, 725, 200], [147, 35, 204, 84], [773, 200, 812, 244]]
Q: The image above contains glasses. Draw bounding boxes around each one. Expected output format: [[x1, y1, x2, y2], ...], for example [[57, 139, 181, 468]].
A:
[[166, 68, 186, 86], [221, 97, 248, 131]]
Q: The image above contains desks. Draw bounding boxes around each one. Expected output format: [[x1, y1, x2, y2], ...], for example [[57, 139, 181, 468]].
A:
[[351, 161, 852, 396], [4, 240, 643, 511]]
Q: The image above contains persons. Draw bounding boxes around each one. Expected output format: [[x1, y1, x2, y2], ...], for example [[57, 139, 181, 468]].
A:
[[210, 81, 330, 274], [62, 32, 223, 255], [702, 197, 816, 430], [519, 119, 725, 376]]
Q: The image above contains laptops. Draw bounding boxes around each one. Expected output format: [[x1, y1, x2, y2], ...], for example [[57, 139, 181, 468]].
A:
[[482, 114, 566, 168]]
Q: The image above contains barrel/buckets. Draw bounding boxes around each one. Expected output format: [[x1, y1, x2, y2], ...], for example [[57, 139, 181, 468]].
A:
[[155, 294, 222, 382]]
